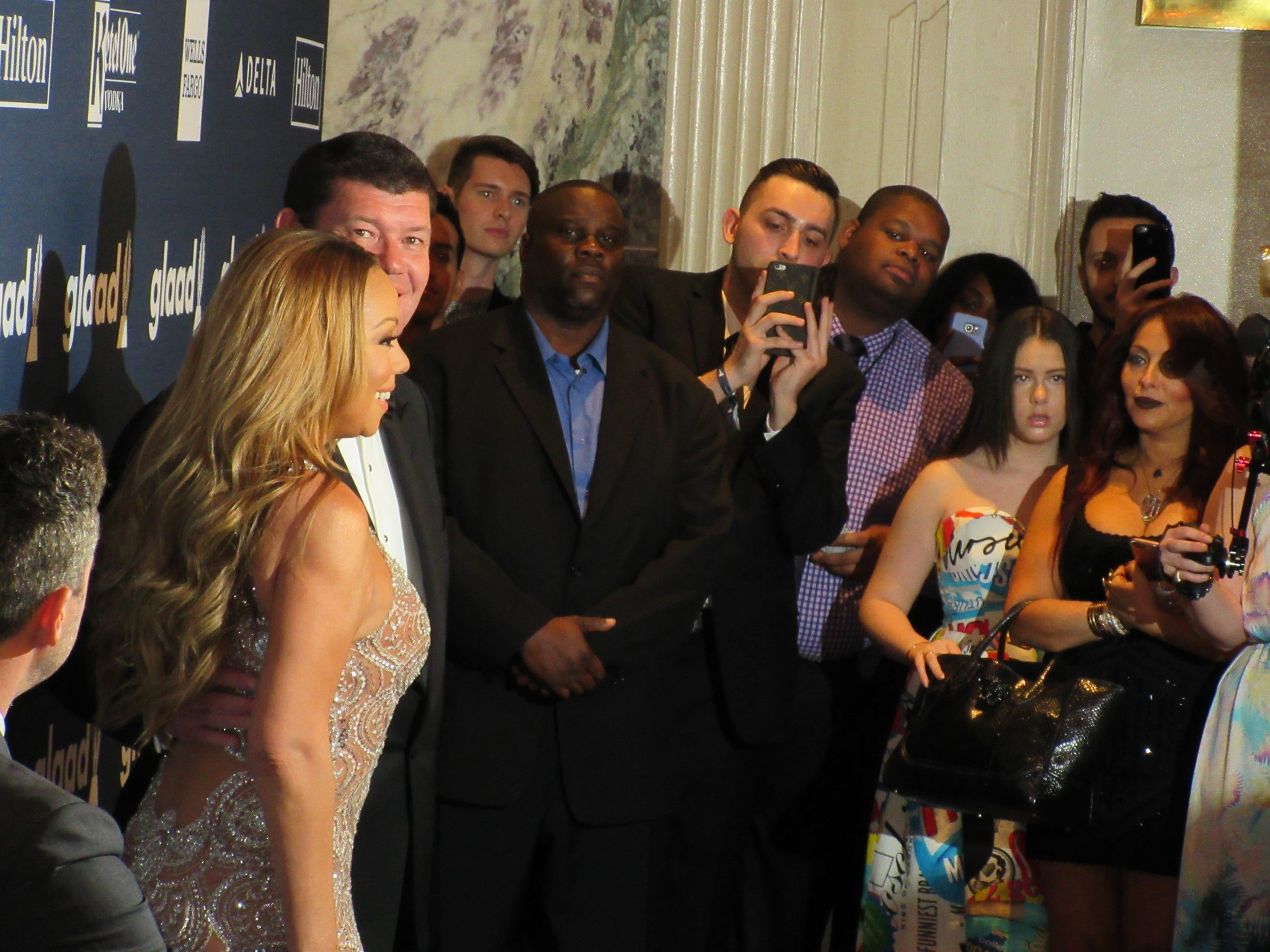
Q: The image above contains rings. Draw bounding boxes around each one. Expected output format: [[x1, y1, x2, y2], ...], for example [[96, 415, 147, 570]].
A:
[[1108, 568, 1125, 580], [1102, 577, 1112, 589], [1172, 568, 1181, 583], [925, 650, 932, 657]]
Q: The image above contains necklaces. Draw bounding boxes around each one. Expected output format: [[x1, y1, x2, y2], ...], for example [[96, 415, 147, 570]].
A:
[[1138, 442, 1188, 478], [1136, 441, 1188, 522]]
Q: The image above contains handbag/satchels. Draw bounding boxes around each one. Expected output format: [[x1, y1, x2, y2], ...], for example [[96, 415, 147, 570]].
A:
[[873, 589, 1122, 838]]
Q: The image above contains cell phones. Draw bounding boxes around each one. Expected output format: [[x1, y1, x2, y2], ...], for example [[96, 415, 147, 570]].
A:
[[1132, 223, 1172, 301], [1130, 537, 1170, 582], [761, 261, 820, 356], [943, 312, 989, 384]]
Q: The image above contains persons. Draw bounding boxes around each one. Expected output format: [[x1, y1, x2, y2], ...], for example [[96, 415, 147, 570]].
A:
[[1075, 192, 1178, 453], [399, 190, 465, 342], [794, 185, 973, 775], [909, 253, 1042, 383], [1159, 438, 1270, 952], [857, 307, 1094, 952], [119, 131, 447, 952], [609, 157, 867, 952], [418, 179, 736, 952], [93, 230, 432, 952], [0, 409, 172, 951], [1003, 291, 1251, 952]]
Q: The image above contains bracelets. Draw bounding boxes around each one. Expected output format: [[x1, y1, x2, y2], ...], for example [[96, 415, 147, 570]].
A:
[[906, 642, 932, 665], [1088, 600, 1129, 638]]
